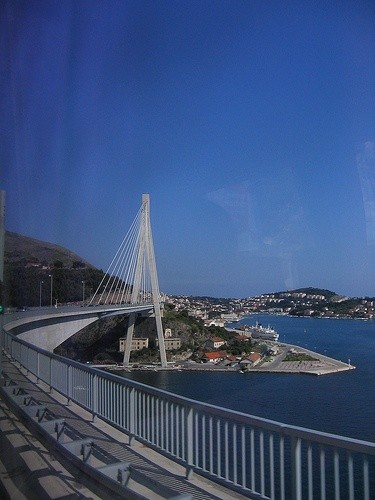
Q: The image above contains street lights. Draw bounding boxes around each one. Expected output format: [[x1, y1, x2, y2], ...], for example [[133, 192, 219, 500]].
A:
[[89, 288, 93, 301], [40, 281, 44, 310], [81, 280, 86, 307], [103, 285, 107, 305], [49, 275, 54, 309]]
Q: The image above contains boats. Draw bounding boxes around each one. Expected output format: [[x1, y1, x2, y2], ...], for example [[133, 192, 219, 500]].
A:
[[237, 323, 278, 344]]
